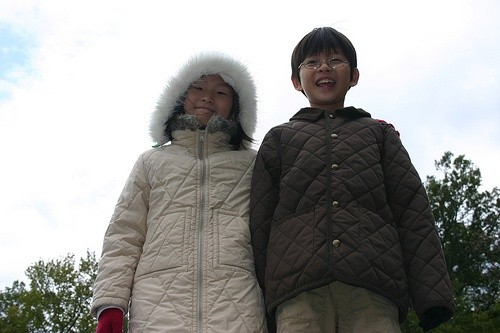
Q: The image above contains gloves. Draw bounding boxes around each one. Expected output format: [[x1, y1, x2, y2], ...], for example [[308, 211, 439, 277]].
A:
[[96, 308, 122, 333], [419, 309, 449, 330]]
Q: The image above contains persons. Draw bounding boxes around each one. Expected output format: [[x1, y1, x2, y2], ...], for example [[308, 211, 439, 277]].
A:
[[90, 52, 269, 333], [250, 27, 455, 333]]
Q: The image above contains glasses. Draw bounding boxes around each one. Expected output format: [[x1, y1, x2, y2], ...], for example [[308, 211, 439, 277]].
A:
[[298, 57, 350, 69]]
